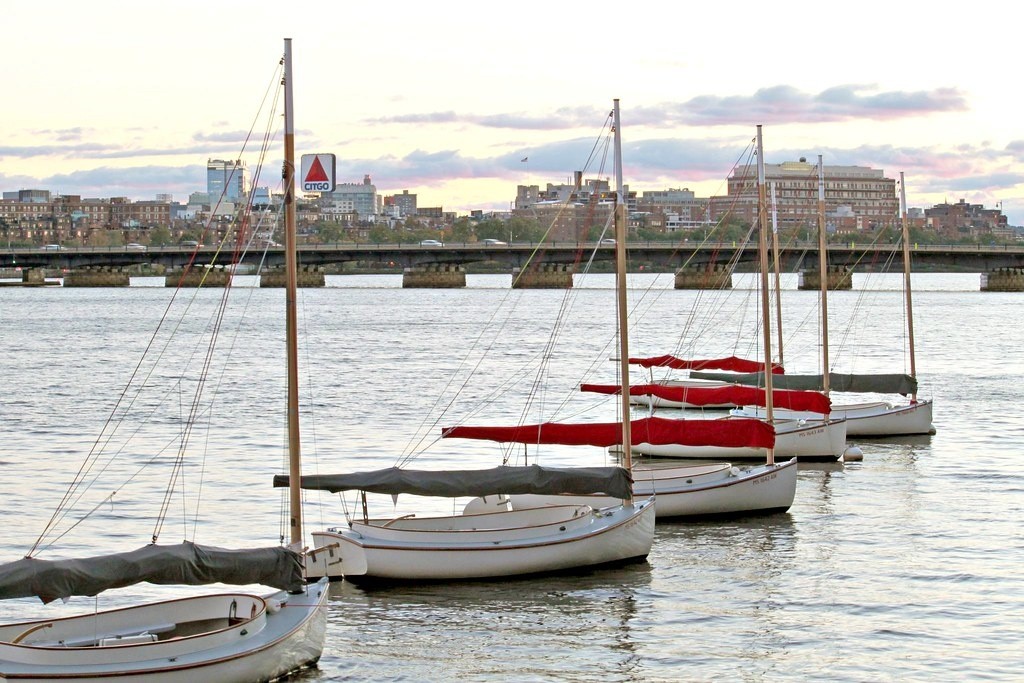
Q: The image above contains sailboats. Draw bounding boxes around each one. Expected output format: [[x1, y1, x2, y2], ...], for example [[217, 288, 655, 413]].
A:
[[723, 172, 936, 437], [0, 37, 345, 683], [444, 156, 847, 461], [457, 125, 797, 520], [581, 156, 888, 413], [274, 99, 657, 582]]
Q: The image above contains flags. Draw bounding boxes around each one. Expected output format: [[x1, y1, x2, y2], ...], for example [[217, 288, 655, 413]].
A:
[[521, 157, 527, 162]]
